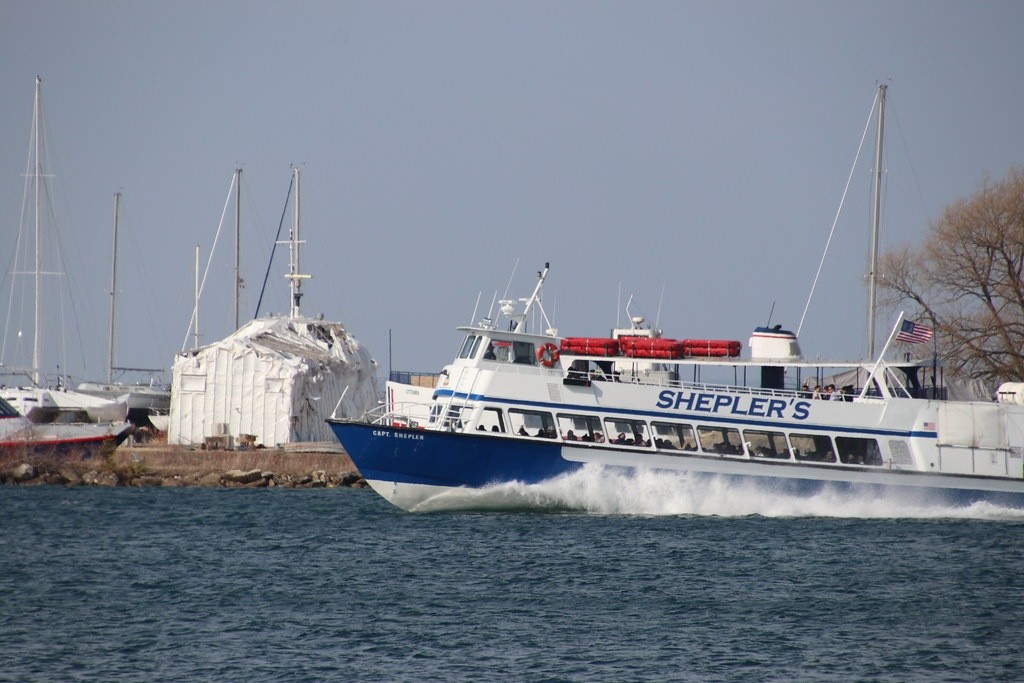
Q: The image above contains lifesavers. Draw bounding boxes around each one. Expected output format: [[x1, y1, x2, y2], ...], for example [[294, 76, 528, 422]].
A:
[[537, 343, 560, 368]]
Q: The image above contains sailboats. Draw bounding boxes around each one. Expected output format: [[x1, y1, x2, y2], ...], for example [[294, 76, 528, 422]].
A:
[[2, 67, 305, 450]]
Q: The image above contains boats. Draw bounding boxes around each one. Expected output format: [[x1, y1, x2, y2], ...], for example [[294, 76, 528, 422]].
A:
[[323, 71, 1024, 524]]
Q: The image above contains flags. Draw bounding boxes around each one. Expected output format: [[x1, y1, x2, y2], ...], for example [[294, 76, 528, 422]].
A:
[[896, 319, 932, 345]]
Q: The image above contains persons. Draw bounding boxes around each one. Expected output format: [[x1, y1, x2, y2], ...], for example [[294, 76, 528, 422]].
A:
[[534, 429, 564, 439], [567, 430, 577, 440], [479, 425, 486, 431], [582, 433, 677, 449], [492, 426, 499, 432], [568, 367, 621, 383], [484, 348, 496, 360], [801, 384, 855, 401], [684, 442, 865, 465], [518, 428, 529, 436]]
